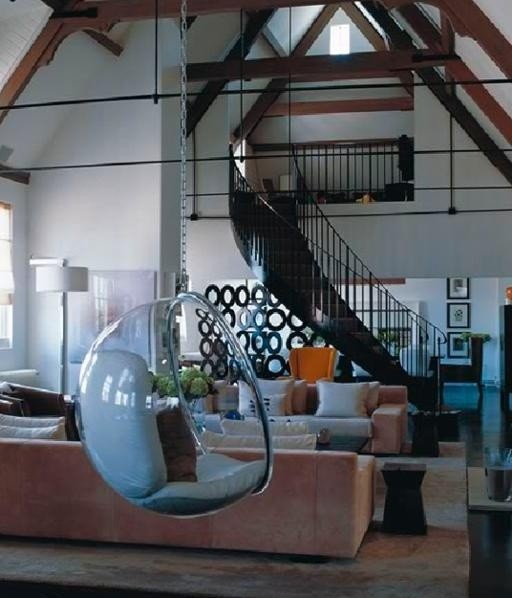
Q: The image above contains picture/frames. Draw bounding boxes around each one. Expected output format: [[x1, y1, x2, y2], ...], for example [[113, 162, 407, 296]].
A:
[[447, 331, 470, 358], [446, 301, 471, 328], [446, 277, 470, 299]]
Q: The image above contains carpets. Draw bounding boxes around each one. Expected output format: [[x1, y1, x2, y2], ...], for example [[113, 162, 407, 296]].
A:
[[0, 438, 472, 598]]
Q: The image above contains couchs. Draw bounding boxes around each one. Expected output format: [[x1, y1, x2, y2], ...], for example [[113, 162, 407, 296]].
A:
[[1, 434, 375, 568], [1, 364, 78, 442], [209, 378, 409, 454]]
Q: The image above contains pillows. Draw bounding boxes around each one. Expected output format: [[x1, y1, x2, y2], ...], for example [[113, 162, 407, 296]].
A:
[[198, 424, 319, 454], [1, 379, 70, 440], [233, 379, 290, 418], [252, 378, 295, 418], [153, 401, 201, 485], [313, 380, 366, 416], [291, 378, 308, 411], [365, 380, 381, 416], [218, 416, 310, 436]]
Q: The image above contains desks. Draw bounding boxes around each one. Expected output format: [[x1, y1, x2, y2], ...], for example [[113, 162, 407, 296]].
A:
[[378, 461, 428, 537], [407, 407, 441, 458]]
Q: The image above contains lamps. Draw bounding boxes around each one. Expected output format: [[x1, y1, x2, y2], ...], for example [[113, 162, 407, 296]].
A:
[[34, 264, 91, 390]]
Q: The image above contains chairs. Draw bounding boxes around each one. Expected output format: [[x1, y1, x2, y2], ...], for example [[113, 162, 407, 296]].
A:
[[289, 347, 337, 380]]
